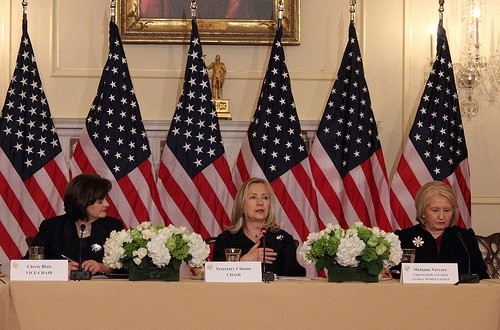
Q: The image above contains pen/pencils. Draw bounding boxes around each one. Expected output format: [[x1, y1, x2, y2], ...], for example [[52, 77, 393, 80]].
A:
[[61, 254, 72, 262]]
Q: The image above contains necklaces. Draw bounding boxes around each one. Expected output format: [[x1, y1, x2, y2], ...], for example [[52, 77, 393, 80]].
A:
[[75, 220, 92, 234], [246, 226, 265, 237]]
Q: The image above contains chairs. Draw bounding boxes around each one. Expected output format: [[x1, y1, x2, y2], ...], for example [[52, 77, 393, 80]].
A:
[[475, 233, 500, 278]]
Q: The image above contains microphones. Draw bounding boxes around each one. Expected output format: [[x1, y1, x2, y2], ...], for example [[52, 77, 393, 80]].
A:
[[454, 232, 479, 283], [70, 224, 92, 281], [259, 228, 274, 281]]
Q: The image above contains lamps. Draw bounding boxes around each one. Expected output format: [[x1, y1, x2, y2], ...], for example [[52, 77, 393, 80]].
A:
[[423, 0, 500, 120]]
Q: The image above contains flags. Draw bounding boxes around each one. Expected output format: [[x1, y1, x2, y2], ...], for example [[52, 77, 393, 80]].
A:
[[68, 21, 155, 232], [309, 20, 389, 277], [231, 25, 318, 280], [0, 20, 70, 277], [389, 23, 472, 230], [156, 18, 232, 263]]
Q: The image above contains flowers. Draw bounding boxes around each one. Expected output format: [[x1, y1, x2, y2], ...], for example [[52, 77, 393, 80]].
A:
[[102, 220, 211, 271], [298, 222, 402, 263]]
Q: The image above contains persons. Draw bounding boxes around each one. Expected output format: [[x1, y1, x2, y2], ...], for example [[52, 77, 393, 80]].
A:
[[387, 181, 491, 280], [21, 174, 125, 276], [211, 178, 306, 278], [204, 54, 226, 99]]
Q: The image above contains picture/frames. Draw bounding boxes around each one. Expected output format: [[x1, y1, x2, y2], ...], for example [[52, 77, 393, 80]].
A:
[[109, 0, 302, 46]]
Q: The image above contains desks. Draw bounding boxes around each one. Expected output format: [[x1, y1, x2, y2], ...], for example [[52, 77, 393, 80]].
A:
[[0, 275, 500, 330]]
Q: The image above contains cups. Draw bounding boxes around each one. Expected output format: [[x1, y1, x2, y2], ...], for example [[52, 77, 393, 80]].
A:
[[401, 249, 416, 263], [225, 248, 242, 261], [28, 246, 44, 260]]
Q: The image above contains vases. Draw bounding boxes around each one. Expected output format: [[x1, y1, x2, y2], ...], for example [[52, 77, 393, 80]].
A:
[[327, 258, 384, 282], [127, 259, 181, 281]]
